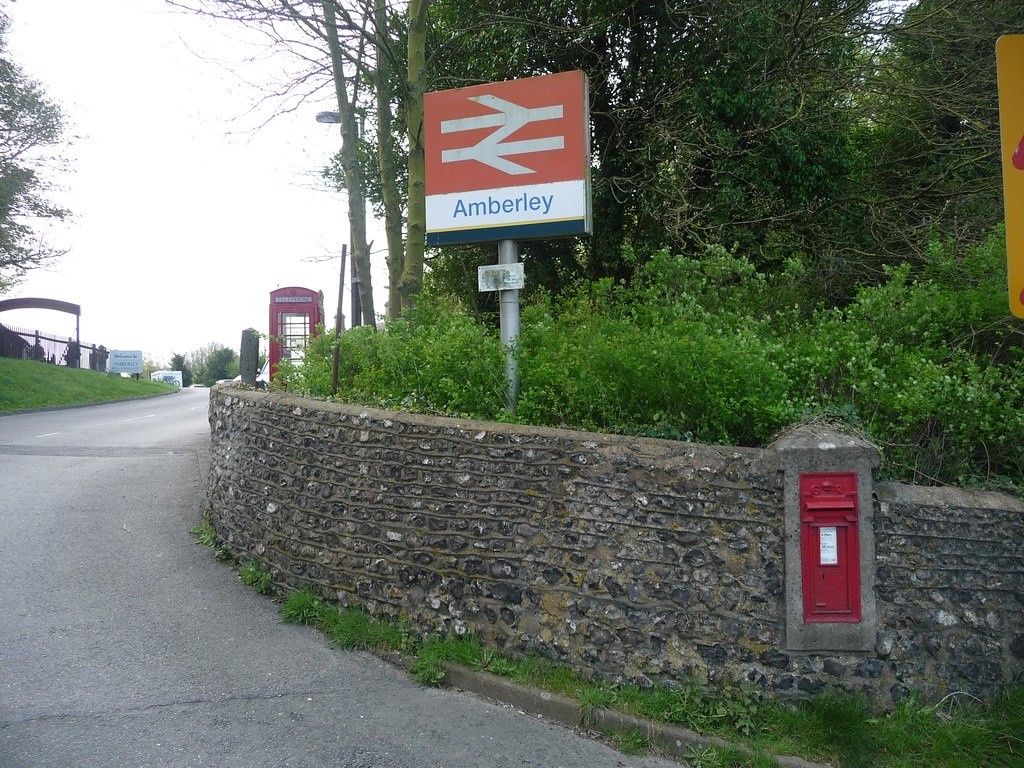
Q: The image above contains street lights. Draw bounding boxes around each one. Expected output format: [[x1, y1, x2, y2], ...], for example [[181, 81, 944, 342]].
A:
[[316, 111, 367, 327]]
